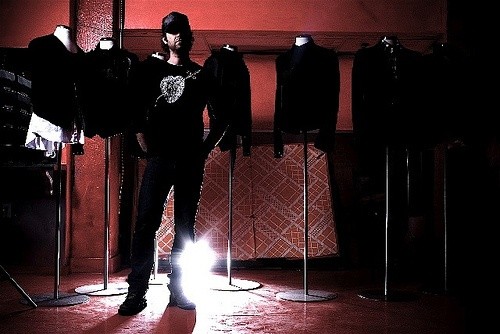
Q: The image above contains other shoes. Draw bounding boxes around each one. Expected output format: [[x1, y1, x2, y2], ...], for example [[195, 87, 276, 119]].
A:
[[118, 291, 148, 316], [170, 292, 196, 310]]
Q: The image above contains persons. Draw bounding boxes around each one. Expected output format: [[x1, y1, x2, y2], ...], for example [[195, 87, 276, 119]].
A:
[[119, 12, 232, 316]]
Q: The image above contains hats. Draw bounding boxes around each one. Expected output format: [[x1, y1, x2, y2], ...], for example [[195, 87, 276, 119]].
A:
[[162, 12, 189, 32]]
